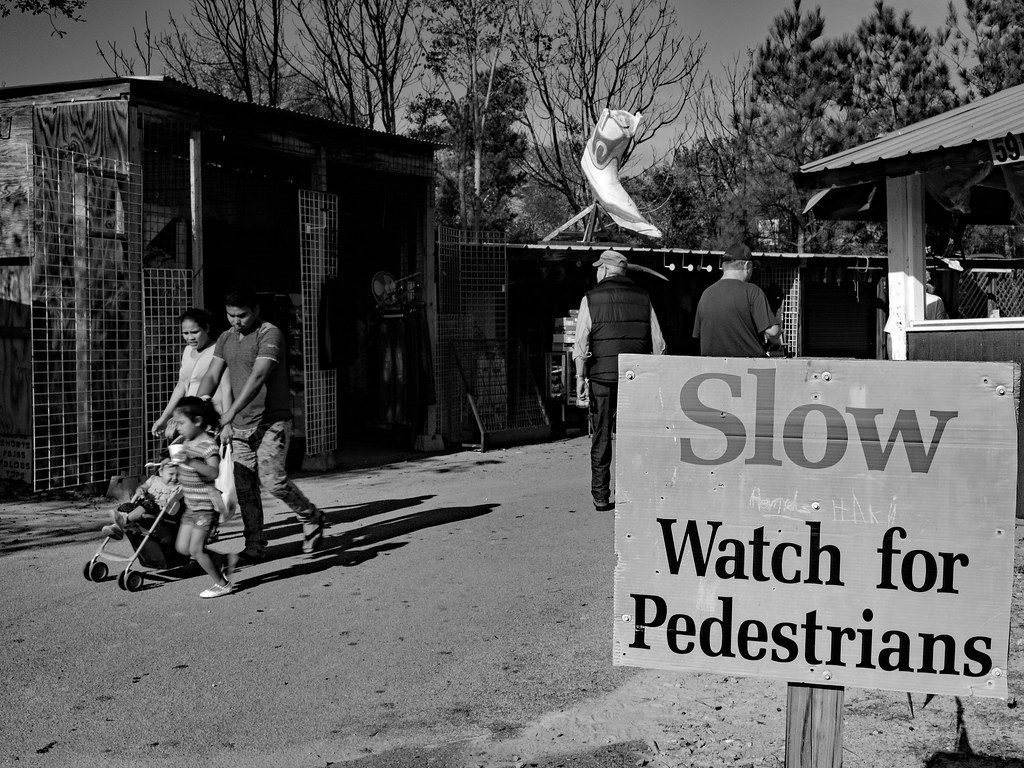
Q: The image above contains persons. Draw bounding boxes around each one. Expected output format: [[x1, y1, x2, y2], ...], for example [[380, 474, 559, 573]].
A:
[[171, 397, 238, 598], [693, 244, 783, 358], [884, 271, 946, 363], [572, 251, 667, 511], [196, 290, 326, 564], [152, 310, 235, 543], [103, 459, 179, 540]]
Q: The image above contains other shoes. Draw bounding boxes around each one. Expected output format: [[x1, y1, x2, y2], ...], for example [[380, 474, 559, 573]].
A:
[[102, 525, 124, 540], [199, 582, 232, 597], [221, 553, 236, 581], [219, 505, 236, 524], [594, 494, 608, 507], [109, 509, 131, 532]]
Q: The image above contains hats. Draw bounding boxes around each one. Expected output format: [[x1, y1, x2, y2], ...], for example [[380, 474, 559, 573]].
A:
[[592, 251, 627, 269], [722, 243, 759, 268]]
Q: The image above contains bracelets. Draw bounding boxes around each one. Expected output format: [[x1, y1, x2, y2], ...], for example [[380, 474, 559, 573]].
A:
[[574, 375, 584, 379]]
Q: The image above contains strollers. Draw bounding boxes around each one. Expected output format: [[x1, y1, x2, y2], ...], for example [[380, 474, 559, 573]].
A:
[[83, 427, 224, 594]]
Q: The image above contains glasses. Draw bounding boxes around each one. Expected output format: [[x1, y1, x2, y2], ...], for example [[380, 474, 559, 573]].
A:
[[596, 266, 609, 271]]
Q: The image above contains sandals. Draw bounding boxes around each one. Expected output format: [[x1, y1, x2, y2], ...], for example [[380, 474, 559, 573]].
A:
[[299, 510, 326, 553], [231, 548, 267, 567]]
